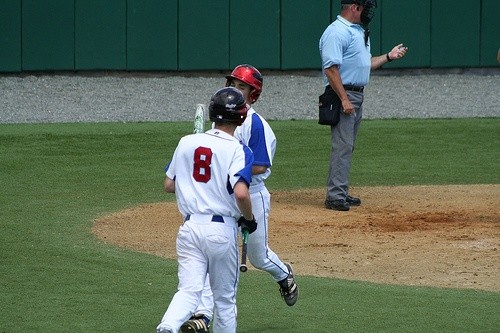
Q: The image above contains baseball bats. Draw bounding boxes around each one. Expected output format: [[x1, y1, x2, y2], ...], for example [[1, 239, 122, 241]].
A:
[[240, 230, 248, 272], [193, 103, 205, 134]]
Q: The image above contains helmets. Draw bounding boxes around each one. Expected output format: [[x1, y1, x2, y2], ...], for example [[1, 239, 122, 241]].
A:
[[225, 63, 263, 103], [209, 86, 247, 125]]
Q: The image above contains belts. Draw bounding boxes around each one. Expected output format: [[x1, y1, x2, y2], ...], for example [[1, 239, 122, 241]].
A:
[[184, 214, 225, 223], [343, 83, 364, 92]]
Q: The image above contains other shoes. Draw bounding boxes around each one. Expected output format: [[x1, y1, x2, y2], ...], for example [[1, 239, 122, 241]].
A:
[[346, 194, 361, 205], [325, 198, 349, 210]]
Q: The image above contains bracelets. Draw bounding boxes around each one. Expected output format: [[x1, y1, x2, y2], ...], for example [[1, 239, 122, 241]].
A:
[[386, 52, 394, 62]]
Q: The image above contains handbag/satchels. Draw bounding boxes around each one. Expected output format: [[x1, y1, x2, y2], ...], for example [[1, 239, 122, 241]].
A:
[[318, 84, 341, 125]]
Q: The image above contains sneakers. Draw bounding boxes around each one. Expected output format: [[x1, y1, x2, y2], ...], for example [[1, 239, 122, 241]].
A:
[[181, 313, 210, 333], [277, 262, 299, 306]]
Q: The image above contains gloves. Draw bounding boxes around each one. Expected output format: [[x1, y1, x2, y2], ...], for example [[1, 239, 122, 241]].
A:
[[238, 213, 257, 234]]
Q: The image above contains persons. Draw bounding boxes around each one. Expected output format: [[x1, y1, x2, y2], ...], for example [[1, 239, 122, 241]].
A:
[[319, 0, 408, 210], [182, 65, 298, 333], [157, 87, 257, 333]]
